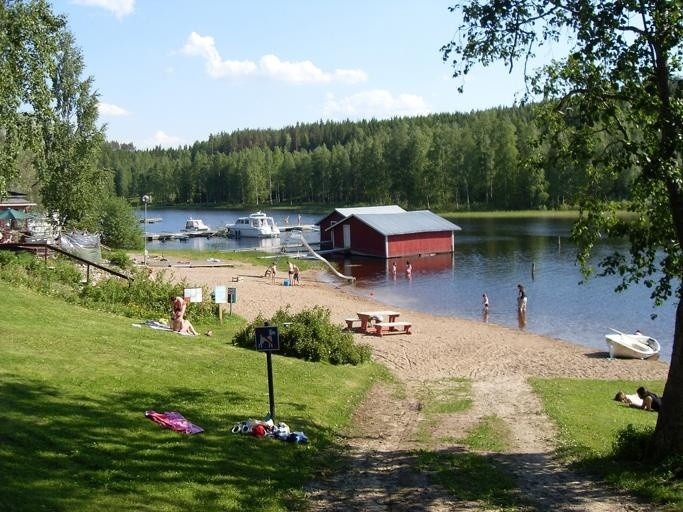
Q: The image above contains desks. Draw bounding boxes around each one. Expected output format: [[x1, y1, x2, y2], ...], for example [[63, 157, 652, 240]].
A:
[[356, 311, 399, 333]]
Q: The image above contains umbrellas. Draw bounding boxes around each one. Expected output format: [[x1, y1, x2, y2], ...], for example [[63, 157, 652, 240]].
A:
[[0, 208, 35, 220]]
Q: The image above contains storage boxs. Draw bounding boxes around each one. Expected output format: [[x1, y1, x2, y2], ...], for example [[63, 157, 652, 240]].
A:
[[283, 279, 290, 286]]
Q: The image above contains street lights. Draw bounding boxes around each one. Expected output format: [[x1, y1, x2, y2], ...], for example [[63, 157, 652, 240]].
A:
[[141, 195, 151, 266], [297, 231, 301, 256]]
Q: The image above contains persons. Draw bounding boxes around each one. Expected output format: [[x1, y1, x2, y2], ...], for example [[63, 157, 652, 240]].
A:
[[482, 285, 528, 315], [168, 294, 197, 334], [615, 393, 642, 406], [392, 261, 398, 281], [406, 260, 412, 279], [270, 260, 300, 284], [629, 387, 662, 411], [485, 313, 525, 332]]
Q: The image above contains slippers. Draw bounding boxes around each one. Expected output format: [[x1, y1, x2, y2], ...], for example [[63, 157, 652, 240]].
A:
[[232, 424, 241, 434], [241, 424, 252, 435]]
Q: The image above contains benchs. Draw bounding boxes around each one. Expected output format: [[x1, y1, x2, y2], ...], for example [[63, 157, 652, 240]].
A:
[[345, 318, 380, 329], [372, 322, 411, 336]]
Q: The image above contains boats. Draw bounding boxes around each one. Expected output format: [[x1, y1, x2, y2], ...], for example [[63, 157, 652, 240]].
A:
[[180, 217, 210, 233], [225, 210, 280, 240], [601, 332, 661, 360]]
